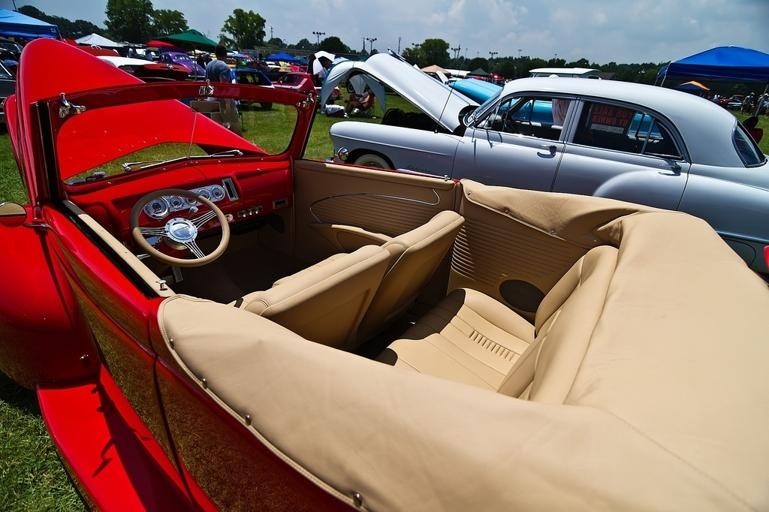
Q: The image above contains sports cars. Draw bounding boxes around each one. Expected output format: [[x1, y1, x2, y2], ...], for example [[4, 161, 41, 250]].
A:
[[0, 37, 769, 512]]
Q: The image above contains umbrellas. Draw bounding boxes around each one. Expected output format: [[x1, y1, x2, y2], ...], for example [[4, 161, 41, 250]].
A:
[[144, 28, 336, 75]]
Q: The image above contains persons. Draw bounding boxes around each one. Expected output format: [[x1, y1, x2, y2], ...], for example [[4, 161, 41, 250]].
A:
[[344, 86, 374, 118], [204, 44, 244, 138], [701, 90, 769, 116]]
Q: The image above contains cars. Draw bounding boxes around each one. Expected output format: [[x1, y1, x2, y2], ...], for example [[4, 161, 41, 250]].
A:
[[0, 39, 23, 123], [62, 39, 369, 109], [320, 53, 769, 280], [448, 77, 662, 141]]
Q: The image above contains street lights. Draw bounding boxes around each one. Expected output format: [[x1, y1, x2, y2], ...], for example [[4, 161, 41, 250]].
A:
[[489, 52, 498, 59], [451, 47, 461, 60], [366, 38, 377, 53], [312, 31, 326, 50]]
